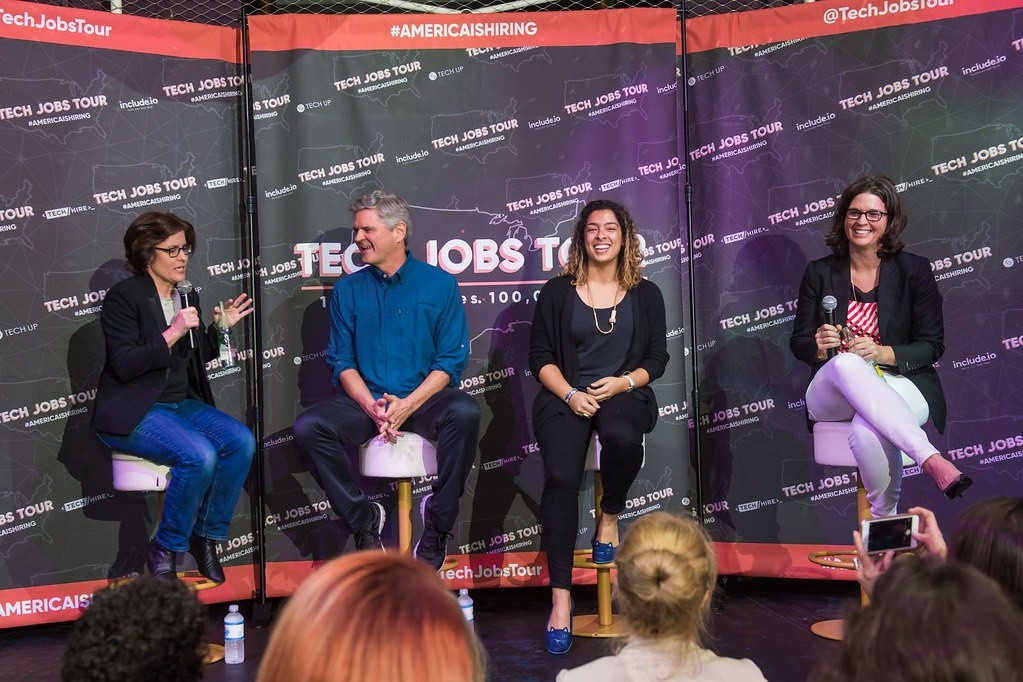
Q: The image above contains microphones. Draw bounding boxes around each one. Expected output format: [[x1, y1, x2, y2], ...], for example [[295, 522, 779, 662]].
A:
[[176, 279, 199, 349], [821, 294, 837, 361]]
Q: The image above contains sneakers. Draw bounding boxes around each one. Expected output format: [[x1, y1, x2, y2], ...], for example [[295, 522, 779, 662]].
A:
[[413, 494, 454, 573], [354, 501, 386, 554]]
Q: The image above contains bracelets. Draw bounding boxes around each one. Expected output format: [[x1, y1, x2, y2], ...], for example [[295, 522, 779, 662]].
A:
[[623, 371, 638, 389], [565, 387, 578, 403]]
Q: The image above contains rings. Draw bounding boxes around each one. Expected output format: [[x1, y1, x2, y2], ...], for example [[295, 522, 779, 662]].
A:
[[865, 349, 868, 355], [854, 557, 863, 569], [583, 411, 589, 418]]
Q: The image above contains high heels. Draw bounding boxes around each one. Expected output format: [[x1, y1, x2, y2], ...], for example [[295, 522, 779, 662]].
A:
[[932, 462, 973, 500]]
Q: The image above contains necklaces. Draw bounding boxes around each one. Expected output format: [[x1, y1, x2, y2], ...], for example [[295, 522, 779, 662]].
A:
[[587, 283, 621, 334], [851, 258, 881, 339]]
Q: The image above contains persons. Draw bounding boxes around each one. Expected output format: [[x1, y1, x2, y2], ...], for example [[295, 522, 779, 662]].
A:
[[835, 558, 1023, 682], [62, 574, 212, 682], [853, 496, 1023, 620], [555, 511, 767, 682], [93, 211, 255, 584], [791, 175, 973, 520], [294, 190, 481, 574], [529, 199, 670, 654], [258, 550, 490, 682]]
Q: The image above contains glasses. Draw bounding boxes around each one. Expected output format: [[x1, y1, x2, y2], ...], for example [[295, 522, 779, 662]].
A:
[[154, 245, 192, 258], [846, 208, 888, 221]]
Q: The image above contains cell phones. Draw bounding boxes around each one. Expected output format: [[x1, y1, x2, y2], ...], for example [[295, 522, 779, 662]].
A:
[[860, 512, 920, 557]]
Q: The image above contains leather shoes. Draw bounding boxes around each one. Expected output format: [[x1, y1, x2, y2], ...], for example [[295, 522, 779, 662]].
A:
[[188, 533, 224, 583], [145, 539, 182, 585]]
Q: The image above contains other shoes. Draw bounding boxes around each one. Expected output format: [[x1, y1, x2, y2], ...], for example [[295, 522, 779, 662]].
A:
[[591, 518, 622, 563], [545, 597, 574, 655]]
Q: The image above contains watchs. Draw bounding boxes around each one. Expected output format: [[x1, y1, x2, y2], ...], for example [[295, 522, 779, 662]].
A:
[[621, 376, 635, 393]]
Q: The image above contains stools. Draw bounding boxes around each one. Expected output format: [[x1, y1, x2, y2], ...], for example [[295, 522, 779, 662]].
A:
[[110, 449, 230, 665], [359, 431, 439, 560], [572, 428, 646, 637], [811, 420, 930, 643]]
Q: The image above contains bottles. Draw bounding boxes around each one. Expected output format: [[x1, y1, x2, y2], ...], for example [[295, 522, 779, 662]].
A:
[[454, 588, 474, 632], [223, 605, 245, 665], [216, 301, 239, 369], [841, 327, 887, 384]]
